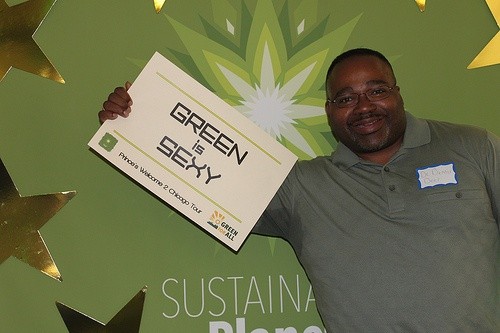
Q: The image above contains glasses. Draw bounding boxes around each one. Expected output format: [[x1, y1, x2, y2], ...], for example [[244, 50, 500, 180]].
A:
[[326, 82, 398, 108]]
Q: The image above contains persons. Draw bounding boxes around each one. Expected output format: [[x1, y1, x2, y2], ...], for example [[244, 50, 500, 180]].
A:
[[99, 46, 500, 333]]
